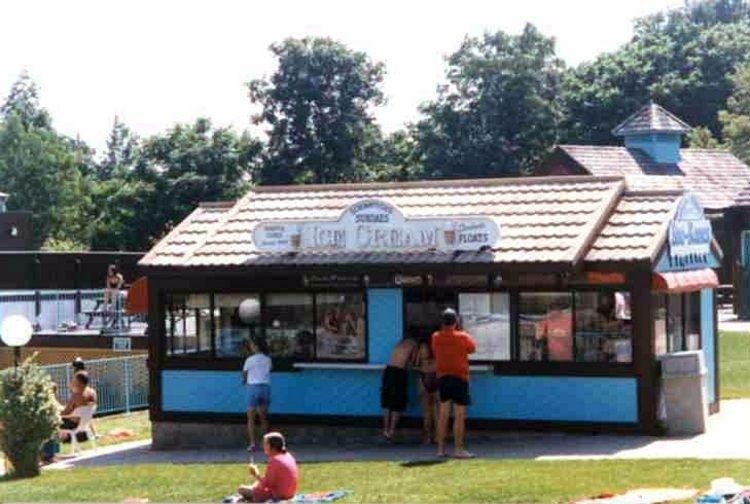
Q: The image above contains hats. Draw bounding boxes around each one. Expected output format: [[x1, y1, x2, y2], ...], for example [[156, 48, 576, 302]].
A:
[[442, 308, 457, 320], [250, 335, 267, 348]]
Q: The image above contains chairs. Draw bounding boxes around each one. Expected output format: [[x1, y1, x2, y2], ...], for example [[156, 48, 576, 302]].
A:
[[57, 403, 100, 456]]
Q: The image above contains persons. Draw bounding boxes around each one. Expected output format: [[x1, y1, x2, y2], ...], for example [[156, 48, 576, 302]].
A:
[[105, 264, 124, 309], [36, 355, 98, 462], [537, 304, 576, 361], [414, 341, 448, 443], [431, 307, 477, 458], [236, 432, 300, 502], [314, 307, 363, 360], [381, 334, 422, 439], [242, 341, 273, 451]]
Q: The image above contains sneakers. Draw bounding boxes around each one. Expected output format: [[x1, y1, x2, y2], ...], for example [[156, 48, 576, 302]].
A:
[[245, 445, 256, 452], [436, 448, 476, 458]]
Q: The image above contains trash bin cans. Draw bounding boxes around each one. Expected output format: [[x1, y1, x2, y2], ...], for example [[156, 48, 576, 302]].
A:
[[659, 351, 708, 437]]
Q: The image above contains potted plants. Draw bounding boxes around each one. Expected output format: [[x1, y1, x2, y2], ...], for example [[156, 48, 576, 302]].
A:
[[0, 359, 61, 476]]
